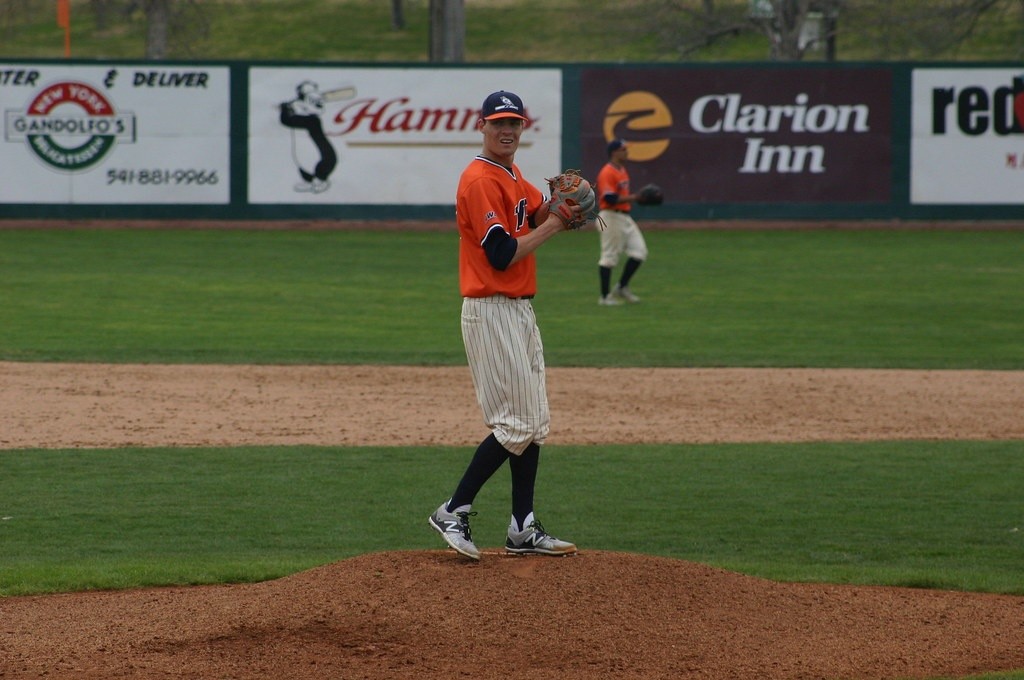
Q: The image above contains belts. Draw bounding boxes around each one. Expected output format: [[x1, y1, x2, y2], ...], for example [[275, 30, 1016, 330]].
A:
[[616, 209, 630, 214], [509, 295, 535, 299]]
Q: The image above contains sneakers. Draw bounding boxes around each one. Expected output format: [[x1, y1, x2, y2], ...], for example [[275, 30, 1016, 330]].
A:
[[428, 502, 481, 560], [505, 521, 578, 556]]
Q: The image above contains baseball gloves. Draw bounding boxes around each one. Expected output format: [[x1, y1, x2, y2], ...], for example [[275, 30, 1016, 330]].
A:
[[639, 183, 664, 206], [546, 173, 596, 230]]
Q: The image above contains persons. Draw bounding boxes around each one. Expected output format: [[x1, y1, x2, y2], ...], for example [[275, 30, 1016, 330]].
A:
[[594, 139, 664, 306], [427, 90, 598, 562]]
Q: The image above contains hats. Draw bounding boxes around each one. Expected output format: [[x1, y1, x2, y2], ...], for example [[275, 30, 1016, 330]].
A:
[[607, 138, 631, 152], [482, 90, 528, 121]]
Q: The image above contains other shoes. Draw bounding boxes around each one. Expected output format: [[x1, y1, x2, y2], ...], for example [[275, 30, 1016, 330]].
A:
[[599, 293, 625, 306], [612, 284, 640, 303]]
